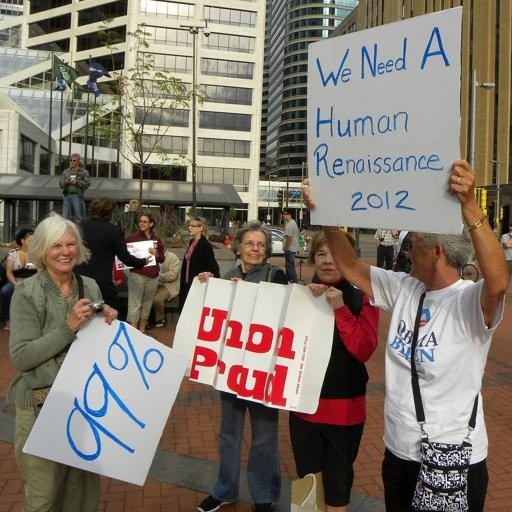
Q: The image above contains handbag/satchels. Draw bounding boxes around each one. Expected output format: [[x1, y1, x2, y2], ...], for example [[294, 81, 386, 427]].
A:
[[412, 442, 472, 512]]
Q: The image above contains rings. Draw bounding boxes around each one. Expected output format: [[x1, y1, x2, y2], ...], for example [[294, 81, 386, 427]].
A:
[[457, 176, 461, 184]]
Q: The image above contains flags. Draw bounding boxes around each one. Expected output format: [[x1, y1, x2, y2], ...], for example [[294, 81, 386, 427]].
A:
[[106, 77, 126, 96], [86, 62, 112, 98], [53, 75, 68, 93], [52, 55, 81, 89], [73, 81, 85, 101]]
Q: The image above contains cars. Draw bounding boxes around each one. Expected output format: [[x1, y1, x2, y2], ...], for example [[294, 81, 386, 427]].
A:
[[268, 226, 307, 254]]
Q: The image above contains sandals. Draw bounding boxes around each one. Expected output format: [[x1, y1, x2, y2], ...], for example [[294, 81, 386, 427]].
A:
[[155, 317, 166, 328]]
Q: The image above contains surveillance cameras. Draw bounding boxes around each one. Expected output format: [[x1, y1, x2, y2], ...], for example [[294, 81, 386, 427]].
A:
[[203, 28, 210, 37]]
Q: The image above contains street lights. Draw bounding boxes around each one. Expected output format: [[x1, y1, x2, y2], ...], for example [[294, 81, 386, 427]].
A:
[[465, 81, 497, 166], [268, 174, 278, 224]]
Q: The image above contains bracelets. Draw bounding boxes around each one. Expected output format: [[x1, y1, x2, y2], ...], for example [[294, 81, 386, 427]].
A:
[[466, 214, 488, 234]]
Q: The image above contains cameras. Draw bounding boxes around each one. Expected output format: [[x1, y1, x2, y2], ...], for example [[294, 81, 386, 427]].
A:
[[88, 300, 105, 313]]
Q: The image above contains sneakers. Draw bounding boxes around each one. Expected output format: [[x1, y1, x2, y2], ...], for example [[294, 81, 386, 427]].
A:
[[197, 496, 233, 512]]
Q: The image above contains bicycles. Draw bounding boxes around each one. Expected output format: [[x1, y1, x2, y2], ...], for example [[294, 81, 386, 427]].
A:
[[460, 264, 479, 283]]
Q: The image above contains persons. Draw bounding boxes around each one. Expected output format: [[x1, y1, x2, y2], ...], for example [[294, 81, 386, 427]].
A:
[[1, 227, 43, 329], [8, 211, 119, 512], [196, 219, 294, 512], [179, 217, 220, 314], [73, 196, 153, 306], [124, 212, 166, 333], [110, 256, 126, 287], [499, 222, 512, 278], [152, 248, 182, 329], [301, 159, 510, 511], [58, 153, 92, 224], [287, 228, 380, 512], [373, 228, 399, 271], [281, 207, 301, 283]]
